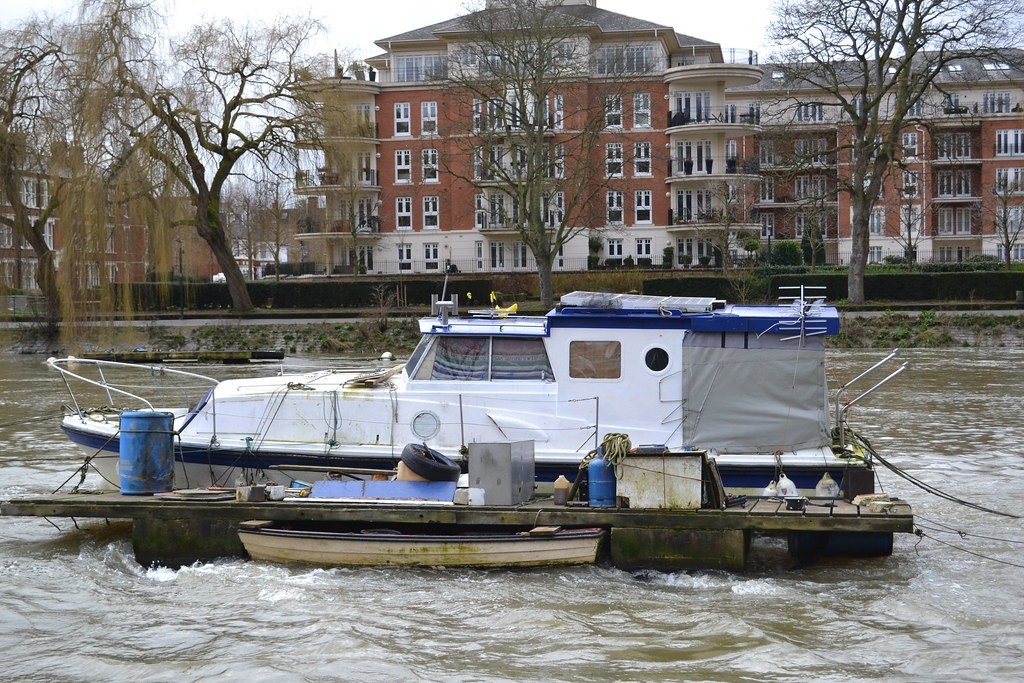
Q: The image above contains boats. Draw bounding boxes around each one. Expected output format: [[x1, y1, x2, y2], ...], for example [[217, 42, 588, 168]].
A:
[[43, 265, 911, 499], [234, 518, 606, 569]]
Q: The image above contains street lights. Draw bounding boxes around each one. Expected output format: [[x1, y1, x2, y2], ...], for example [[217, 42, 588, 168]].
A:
[[174, 237, 185, 320]]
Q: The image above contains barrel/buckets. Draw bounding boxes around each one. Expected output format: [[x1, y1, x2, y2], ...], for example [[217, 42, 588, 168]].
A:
[[120, 412, 174, 494]]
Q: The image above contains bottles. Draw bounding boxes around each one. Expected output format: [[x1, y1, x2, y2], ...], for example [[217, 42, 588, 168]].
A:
[[588, 447, 616, 507], [553, 475, 570, 505]]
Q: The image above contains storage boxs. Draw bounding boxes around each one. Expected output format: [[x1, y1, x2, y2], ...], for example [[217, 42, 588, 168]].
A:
[[616, 455, 703, 509]]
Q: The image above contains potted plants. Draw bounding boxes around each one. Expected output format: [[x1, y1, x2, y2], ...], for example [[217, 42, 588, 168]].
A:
[[681, 255, 693, 268], [358, 264, 367, 274]]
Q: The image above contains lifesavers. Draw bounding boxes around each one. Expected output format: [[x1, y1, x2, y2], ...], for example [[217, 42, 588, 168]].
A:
[[400, 443, 461, 482]]
